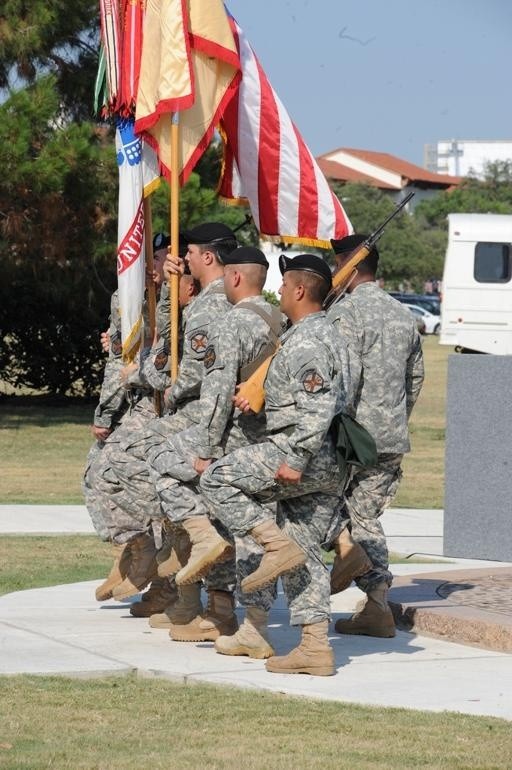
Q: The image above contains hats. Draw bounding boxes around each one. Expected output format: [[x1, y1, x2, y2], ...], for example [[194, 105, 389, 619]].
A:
[[153, 223, 269, 275], [330, 235, 379, 259], [279, 255, 332, 286]]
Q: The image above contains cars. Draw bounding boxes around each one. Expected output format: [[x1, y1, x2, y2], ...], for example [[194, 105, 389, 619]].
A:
[[385, 291, 440, 337]]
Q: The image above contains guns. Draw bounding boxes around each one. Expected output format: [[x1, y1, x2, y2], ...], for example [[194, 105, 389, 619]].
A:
[[235, 192, 416, 414]]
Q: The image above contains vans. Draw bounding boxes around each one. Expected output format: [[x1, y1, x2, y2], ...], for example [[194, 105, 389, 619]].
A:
[[438, 210, 511, 357]]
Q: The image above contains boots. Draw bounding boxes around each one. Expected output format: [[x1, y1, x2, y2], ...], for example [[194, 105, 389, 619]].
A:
[[96, 513, 335, 677], [330, 527, 374, 595], [335, 582, 396, 637]]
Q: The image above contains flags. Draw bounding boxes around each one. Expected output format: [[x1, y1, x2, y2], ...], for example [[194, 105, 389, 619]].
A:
[[134, 0, 243, 186], [116, 116, 163, 364], [213, 2, 355, 248], [94, 0, 145, 125]]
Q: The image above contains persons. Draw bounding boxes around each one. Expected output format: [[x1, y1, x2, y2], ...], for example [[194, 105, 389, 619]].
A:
[[82, 233, 201, 628], [328, 233, 425, 639], [147, 247, 293, 659], [199, 255, 377, 675], [105, 223, 239, 641], [374, 279, 442, 295]]
